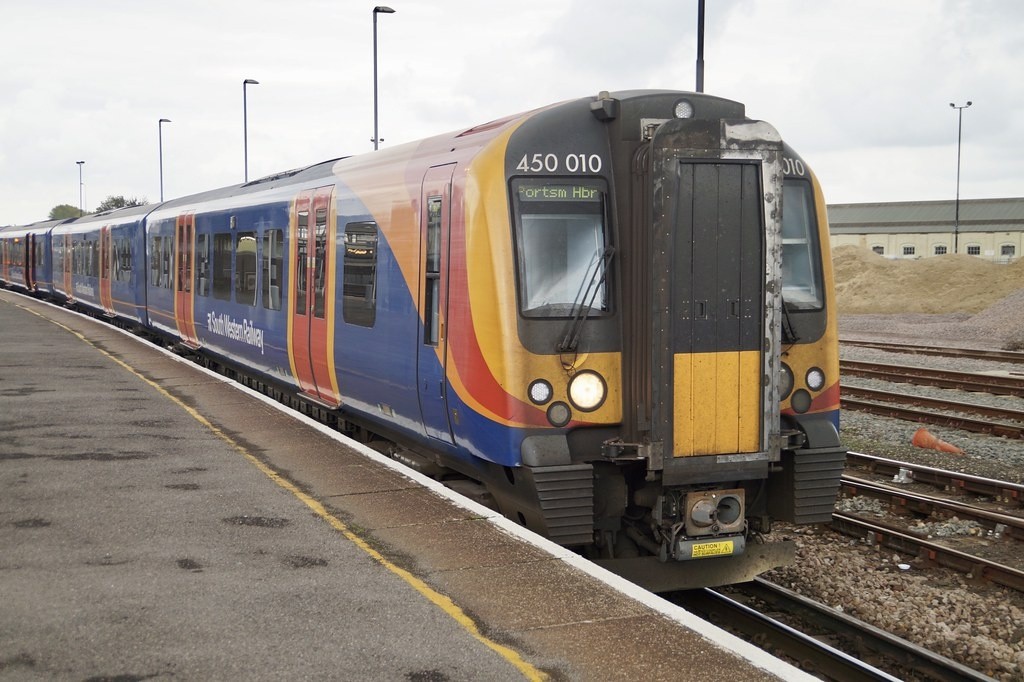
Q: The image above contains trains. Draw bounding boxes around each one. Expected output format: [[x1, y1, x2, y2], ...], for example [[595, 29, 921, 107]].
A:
[[0, 89, 844, 589]]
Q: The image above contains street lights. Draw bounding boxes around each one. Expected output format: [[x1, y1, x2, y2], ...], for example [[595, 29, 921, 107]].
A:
[[159, 119, 171, 202], [371, 6, 395, 151], [243, 80, 259, 183]]
